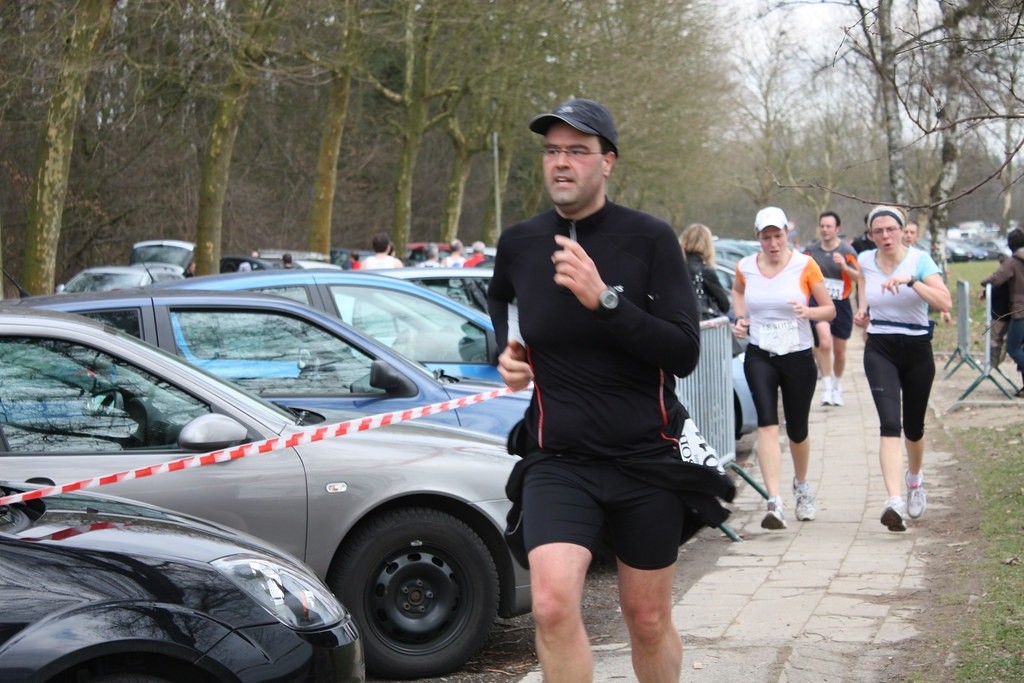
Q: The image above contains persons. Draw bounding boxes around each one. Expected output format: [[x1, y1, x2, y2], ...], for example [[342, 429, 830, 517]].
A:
[[488, 101, 701, 683], [237, 263, 252, 272], [182, 262, 197, 279], [850, 206, 953, 532], [414, 238, 487, 270], [801, 212, 861, 406], [979, 229, 1024, 396], [732, 206, 837, 530], [352, 234, 406, 271], [278, 254, 297, 270], [676, 222, 730, 351]]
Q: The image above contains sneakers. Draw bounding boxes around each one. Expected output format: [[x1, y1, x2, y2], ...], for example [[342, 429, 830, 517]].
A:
[[906, 470, 926, 519], [881, 503, 907, 532], [832, 388, 842, 406], [820, 386, 832, 405], [793, 476, 814, 521], [760, 501, 786, 529]]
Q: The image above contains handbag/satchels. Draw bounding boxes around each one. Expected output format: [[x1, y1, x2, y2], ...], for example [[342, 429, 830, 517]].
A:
[[695, 264, 722, 321]]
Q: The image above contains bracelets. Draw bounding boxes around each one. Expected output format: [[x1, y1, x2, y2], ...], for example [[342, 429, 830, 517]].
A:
[[735, 315, 747, 326], [908, 275, 919, 287]]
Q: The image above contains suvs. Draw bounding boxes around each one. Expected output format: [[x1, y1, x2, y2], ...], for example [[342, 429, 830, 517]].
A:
[[946, 227, 1000, 239]]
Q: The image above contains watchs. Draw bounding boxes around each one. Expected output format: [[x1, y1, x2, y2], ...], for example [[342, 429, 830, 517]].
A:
[[595, 285, 620, 317]]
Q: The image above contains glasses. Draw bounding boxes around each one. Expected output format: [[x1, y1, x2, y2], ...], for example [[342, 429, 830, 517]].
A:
[[539, 144, 608, 161], [871, 227, 900, 237]]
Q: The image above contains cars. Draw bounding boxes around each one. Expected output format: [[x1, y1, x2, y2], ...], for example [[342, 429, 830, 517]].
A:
[[916, 239, 1013, 263], [0, 304, 535, 677], [711, 236, 762, 440], [0, 288, 537, 440], [0, 480, 364, 683], [51, 239, 520, 335]]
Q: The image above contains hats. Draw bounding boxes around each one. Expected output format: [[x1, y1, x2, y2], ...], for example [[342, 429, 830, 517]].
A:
[[1008, 230, 1024, 250], [755, 206, 788, 232], [529, 98, 619, 154], [868, 205, 905, 229]]
